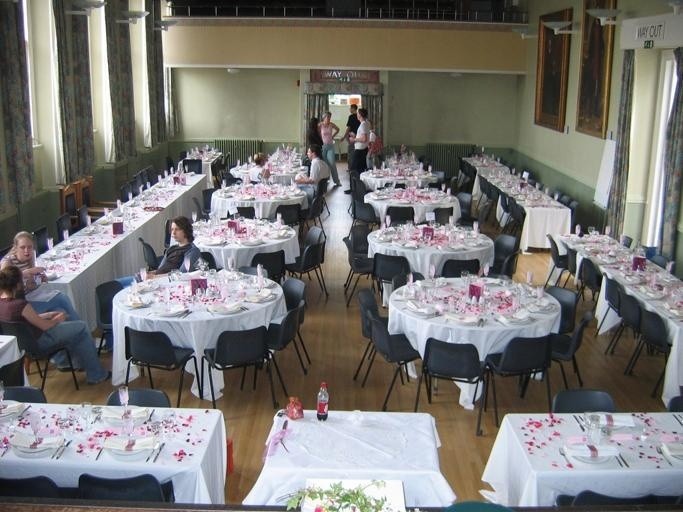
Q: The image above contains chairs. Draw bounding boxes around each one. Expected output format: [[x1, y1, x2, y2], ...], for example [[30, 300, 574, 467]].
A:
[[179, 151, 187, 161], [140, 168, 150, 190], [239, 266, 268, 279], [545, 286, 577, 334], [0, 246, 13, 259], [558, 195, 570, 206], [0, 350, 25, 386], [624, 305, 671, 397], [300, 225, 323, 281], [429, 171, 445, 189], [202, 189, 218, 215], [372, 253, 410, 309], [414, 337, 498, 437], [552, 390, 615, 413], [166, 157, 175, 175], [347, 199, 381, 239], [544, 234, 568, 288], [283, 277, 305, 312], [385, 206, 414, 227], [484, 334, 552, 428], [459, 163, 477, 193], [649, 255, 669, 270], [164, 218, 172, 255], [304, 158, 311, 176], [668, 395, 683, 412], [286, 243, 329, 296], [32, 226, 48, 257], [134, 172, 143, 192], [581, 213, 604, 235], [211, 155, 224, 186], [533, 311, 592, 390], [355, 177, 366, 203], [385, 183, 406, 189], [76, 205, 87, 229], [577, 258, 603, 313], [0, 320, 78, 391], [226, 172, 242, 187], [56, 213, 72, 241], [60, 183, 103, 230], [343, 236, 383, 307], [551, 190, 562, 201], [0, 476, 58, 498], [15, 291, 25, 300], [227, 207, 255, 219], [3, 387, 47, 403], [496, 192, 525, 236], [314, 177, 330, 215], [348, 170, 360, 213], [302, 196, 327, 238], [78, 473, 165, 502], [192, 197, 210, 221], [442, 259, 480, 278], [350, 225, 372, 253], [617, 235, 633, 248], [604, 286, 641, 354], [458, 157, 470, 183], [556, 490, 679, 507], [361, 309, 422, 411], [433, 207, 453, 226], [220, 152, 231, 179], [595, 273, 624, 354], [521, 168, 535, 185], [251, 249, 286, 284], [495, 157, 513, 174], [555, 240, 577, 288], [485, 182, 499, 225], [201, 326, 278, 409], [261, 300, 311, 398], [138, 237, 164, 272], [275, 203, 302, 241], [353, 288, 388, 380], [392, 272, 425, 292], [128, 180, 139, 197], [107, 388, 171, 408], [481, 249, 520, 280], [456, 192, 473, 216], [78, 176, 117, 213], [120, 184, 129, 203], [476, 174, 487, 210], [530, 180, 543, 191], [494, 234, 518, 265], [96, 281, 124, 356], [183, 159, 202, 174], [124, 326, 202, 408], [146, 165, 158, 186], [456, 201, 493, 232], [418, 155, 431, 171], [567, 201, 579, 233], [200, 252, 222, 272]]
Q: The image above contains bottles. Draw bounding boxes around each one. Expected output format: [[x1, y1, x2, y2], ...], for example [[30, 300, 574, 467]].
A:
[[317, 382, 329, 421]]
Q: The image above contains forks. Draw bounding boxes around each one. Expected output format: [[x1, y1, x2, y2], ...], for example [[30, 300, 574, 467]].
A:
[[656, 446, 672, 465], [558, 447, 570, 464]]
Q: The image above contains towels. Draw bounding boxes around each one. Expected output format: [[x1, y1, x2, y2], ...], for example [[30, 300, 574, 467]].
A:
[[416, 277, 447, 287], [129, 293, 142, 307], [13, 433, 64, 447], [564, 444, 619, 456], [500, 309, 530, 323], [209, 302, 244, 314], [105, 435, 155, 449], [250, 288, 273, 303], [407, 299, 439, 315], [153, 304, 185, 315], [663, 302, 683, 317], [587, 412, 635, 426], [640, 285, 657, 298], [1, 403, 25, 416], [445, 312, 478, 323], [104, 408, 150, 419]]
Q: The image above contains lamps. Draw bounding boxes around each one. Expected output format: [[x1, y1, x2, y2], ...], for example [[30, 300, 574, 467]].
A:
[[513, 28, 537, 40], [116, 11, 149, 24], [586, 9, 622, 26], [154, 21, 178, 31], [665, 0, 683, 16], [543, 22, 576, 34], [228, 68, 239, 74], [65, 0, 104, 15], [449, 72, 462, 78]]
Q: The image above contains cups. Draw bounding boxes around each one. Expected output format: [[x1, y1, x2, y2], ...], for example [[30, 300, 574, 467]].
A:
[[164, 171, 168, 177], [374, 183, 377, 191], [420, 162, 423, 169], [192, 211, 197, 223], [554, 191, 558, 200], [140, 267, 147, 281], [483, 264, 489, 280], [385, 215, 391, 229], [382, 161, 385, 169], [544, 186, 549, 194], [406, 273, 413, 288], [117, 200, 120, 207], [48, 238, 53, 252], [588, 226, 595, 237], [104, 208, 108, 216], [118, 386, 129, 410], [447, 187, 451, 197], [461, 270, 469, 289], [86, 216, 91, 226], [372, 165, 376, 171], [449, 216, 454, 225], [210, 269, 216, 275], [147, 182, 150, 189], [234, 213, 240, 219], [473, 221, 479, 232], [129, 192, 132, 200], [277, 213, 282, 223], [0, 382, 6, 405], [391, 180, 396, 188], [170, 168, 173, 174], [253, 216, 259, 221], [221, 179, 227, 188], [64, 230, 69, 241], [417, 179, 422, 188], [428, 164, 433, 173], [526, 271, 533, 285], [537, 284, 544, 299], [195, 287, 205, 299], [441, 183, 446, 192], [591, 230, 599, 240], [423, 185, 429, 191], [646, 270, 656, 287], [428, 264, 435, 282], [535, 182, 539, 190], [158, 175, 161, 182]]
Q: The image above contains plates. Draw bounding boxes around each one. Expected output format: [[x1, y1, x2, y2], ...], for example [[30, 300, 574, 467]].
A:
[[9, 435, 51, 453], [102, 406, 149, 427], [246, 295, 276, 303], [568, 442, 616, 463], [151, 305, 186, 317], [104, 435, 152, 459], [242, 241, 262, 246], [240, 198, 254, 201], [206, 241, 226, 246], [662, 442, 682, 461], [208, 305, 239, 314], [272, 196, 289, 199]]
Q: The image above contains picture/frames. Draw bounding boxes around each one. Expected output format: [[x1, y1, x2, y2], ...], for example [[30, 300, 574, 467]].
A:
[[534, 7, 573, 133], [575, 0, 617, 140]]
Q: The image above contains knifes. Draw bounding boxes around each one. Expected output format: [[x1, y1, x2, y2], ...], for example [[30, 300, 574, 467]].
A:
[[152, 442, 166, 463], [56, 439, 73, 460]]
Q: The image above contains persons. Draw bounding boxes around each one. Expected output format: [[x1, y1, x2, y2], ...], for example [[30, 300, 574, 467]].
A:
[[367, 120, 377, 170], [341, 104, 360, 172], [308, 118, 324, 160], [294, 145, 330, 203], [317, 112, 342, 186], [0, 231, 79, 322], [344, 108, 370, 195], [105, 216, 199, 354], [0, 266, 111, 385]]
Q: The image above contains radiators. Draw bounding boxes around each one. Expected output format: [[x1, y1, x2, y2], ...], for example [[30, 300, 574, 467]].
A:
[[214, 139, 263, 172], [425, 143, 478, 180]]
